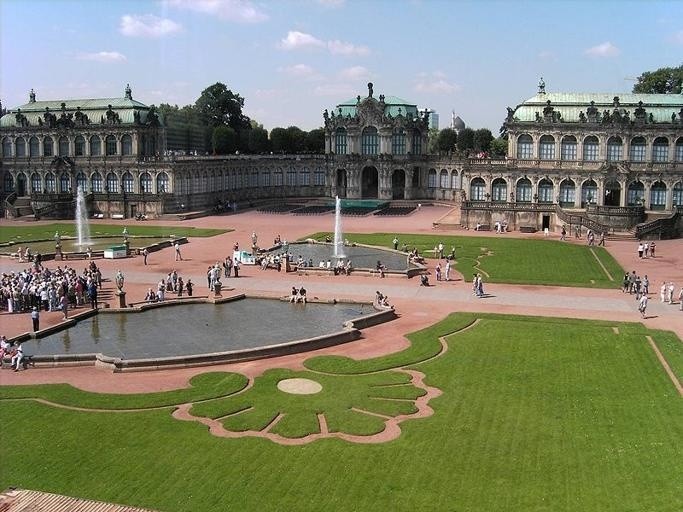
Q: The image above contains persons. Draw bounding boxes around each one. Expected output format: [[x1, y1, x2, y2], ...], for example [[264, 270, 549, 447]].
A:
[[375, 261, 387, 278], [174, 241, 183, 261], [232, 242, 240, 251], [0, 245, 102, 332], [290, 286, 298, 304], [257, 251, 354, 276], [477, 274, 484, 296], [638, 242, 658, 258], [376, 290, 384, 300], [326, 237, 333, 243], [0, 336, 10, 366], [207, 256, 242, 291], [622, 271, 683, 318], [555, 224, 607, 247], [274, 235, 281, 243], [382, 295, 389, 307], [472, 273, 478, 296], [392, 237, 456, 281], [145, 270, 195, 303], [11, 340, 24, 372], [296, 287, 307, 305], [143, 248, 150, 266]]
